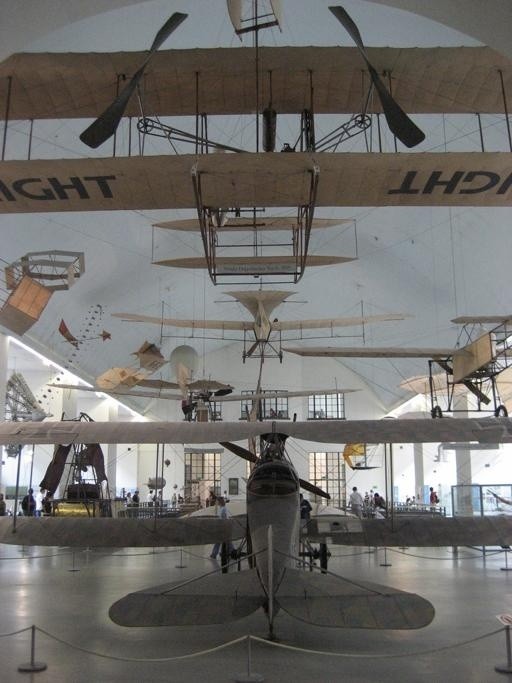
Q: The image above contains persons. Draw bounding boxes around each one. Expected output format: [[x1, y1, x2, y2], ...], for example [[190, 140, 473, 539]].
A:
[[409, 495, 416, 504], [42, 491, 53, 515], [36, 487, 46, 516], [429, 486, 439, 511], [209, 496, 235, 558], [364, 490, 385, 508], [300, 492, 312, 519], [21, 487, 37, 516], [348, 486, 364, 516], [126, 489, 229, 508]]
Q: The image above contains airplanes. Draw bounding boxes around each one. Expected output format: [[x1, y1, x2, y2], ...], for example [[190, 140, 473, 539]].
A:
[[0, 416, 512, 642], [0, 0, 512, 426]]
